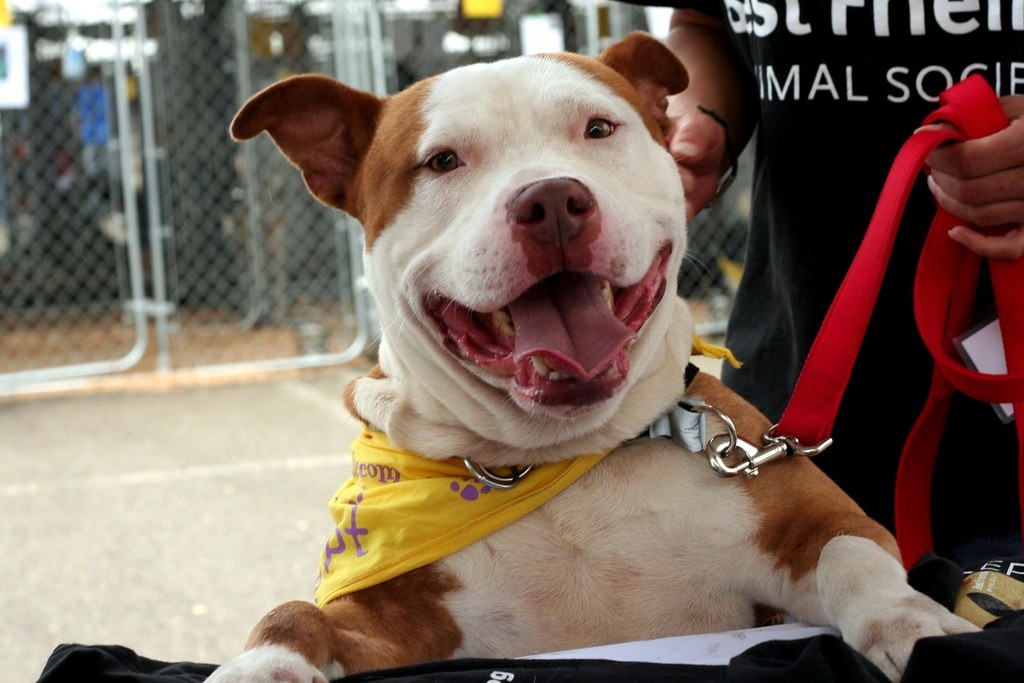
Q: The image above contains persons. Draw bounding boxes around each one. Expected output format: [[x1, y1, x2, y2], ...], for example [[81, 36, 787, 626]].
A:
[[662, 0, 1024, 568]]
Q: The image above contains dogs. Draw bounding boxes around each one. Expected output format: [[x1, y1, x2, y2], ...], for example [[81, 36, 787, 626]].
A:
[[201, 29, 986, 682]]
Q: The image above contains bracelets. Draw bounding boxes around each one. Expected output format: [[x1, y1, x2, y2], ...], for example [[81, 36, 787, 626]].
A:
[[695, 103, 738, 209]]
